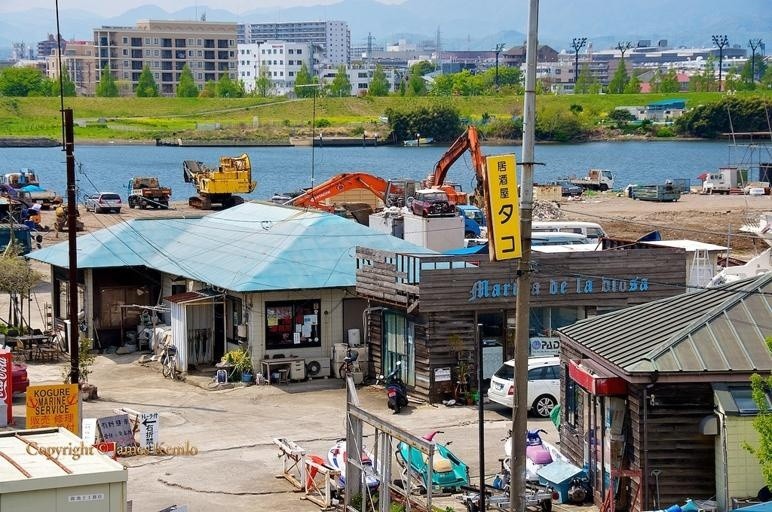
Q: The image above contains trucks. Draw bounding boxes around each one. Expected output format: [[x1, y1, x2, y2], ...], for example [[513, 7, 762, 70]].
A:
[[517, 183, 562, 209], [557, 169, 618, 191], [123, 176, 173, 209], [624, 178, 690, 202]]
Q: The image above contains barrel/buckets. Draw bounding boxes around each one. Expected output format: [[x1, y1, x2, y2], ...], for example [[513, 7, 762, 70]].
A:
[[347, 330, 361, 347]]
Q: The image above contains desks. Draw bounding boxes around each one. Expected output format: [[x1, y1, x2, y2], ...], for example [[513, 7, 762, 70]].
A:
[[16, 335, 49, 361], [260, 357, 305, 385]]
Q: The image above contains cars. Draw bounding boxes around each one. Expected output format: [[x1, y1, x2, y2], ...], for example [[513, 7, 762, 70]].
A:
[[85, 191, 123, 213], [5, 168, 63, 210], [11, 361, 29, 402]]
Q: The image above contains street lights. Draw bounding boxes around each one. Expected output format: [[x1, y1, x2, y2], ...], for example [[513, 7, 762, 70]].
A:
[[615, 41, 630, 93], [711, 34, 727, 93], [494, 44, 504, 93]]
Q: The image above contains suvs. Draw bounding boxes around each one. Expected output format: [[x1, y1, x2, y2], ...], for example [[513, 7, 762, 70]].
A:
[[554, 179, 583, 197], [488, 356, 563, 417]]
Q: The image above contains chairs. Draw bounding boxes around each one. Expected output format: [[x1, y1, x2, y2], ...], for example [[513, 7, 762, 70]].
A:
[[15, 338, 60, 367]]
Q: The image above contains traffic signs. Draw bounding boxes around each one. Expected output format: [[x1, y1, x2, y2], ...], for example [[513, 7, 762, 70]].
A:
[[572, 36, 588, 84]]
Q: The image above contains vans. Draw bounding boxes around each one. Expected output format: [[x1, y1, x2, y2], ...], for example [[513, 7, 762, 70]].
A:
[[531, 219, 609, 252]]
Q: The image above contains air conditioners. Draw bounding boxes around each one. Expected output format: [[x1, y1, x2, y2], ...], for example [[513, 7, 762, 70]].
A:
[[305, 356, 332, 378]]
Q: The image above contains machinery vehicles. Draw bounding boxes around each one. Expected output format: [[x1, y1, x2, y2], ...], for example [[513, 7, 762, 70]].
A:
[[183, 153, 256, 210], [286, 124, 483, 236]]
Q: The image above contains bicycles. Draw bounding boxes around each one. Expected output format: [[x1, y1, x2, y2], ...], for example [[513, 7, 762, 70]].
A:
[[339, 342, 359, 381], [160, 343, 179, 382]]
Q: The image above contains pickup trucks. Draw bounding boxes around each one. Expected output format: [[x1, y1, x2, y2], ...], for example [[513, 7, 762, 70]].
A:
[[703, 173, 731, 194]]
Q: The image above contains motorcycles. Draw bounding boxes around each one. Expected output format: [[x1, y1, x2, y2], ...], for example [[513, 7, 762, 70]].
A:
[[499, 428, 590, 505], [376, 361, 409, 414], [397, 430, 472, 495], [329, 436, 381, 494]]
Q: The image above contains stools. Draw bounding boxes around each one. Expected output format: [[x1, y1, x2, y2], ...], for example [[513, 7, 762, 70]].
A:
[[278, 369, 288, 386]]
